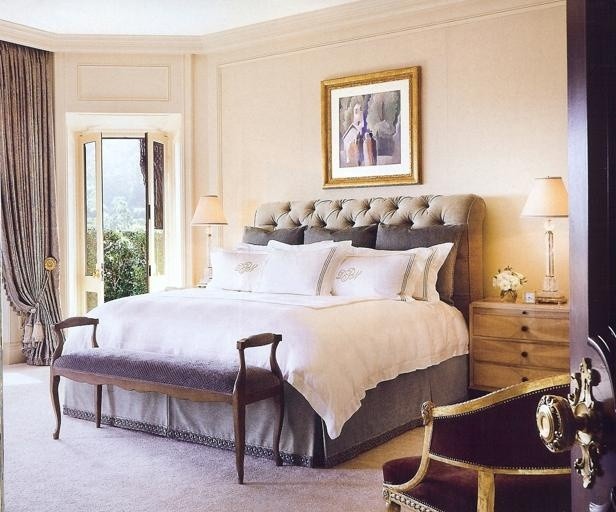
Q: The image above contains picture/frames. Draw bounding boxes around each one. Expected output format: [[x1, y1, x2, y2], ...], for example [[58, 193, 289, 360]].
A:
[[320, 65, 422, 191], [523, 290, 536, 304]]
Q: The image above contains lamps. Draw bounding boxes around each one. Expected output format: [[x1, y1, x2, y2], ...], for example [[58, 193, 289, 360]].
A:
[[521, 178, 570, 304], [190, 195, 229, 288]]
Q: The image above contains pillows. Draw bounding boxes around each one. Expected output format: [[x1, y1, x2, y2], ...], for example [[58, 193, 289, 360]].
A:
[[242, 226, 307, 245], [375, 223, 465, 304], [205, 240, 454, 303], [303, 225, 378, 249]]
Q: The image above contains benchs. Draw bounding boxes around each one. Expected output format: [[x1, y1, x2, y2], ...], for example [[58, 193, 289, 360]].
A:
[[49, 313, 286, 486]]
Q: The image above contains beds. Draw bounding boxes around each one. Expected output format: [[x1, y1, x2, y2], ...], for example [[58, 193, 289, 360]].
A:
[[62, 194, 487, 470]]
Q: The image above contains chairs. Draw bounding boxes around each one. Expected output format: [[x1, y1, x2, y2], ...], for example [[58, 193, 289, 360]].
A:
[[379, 368, 570, 510]]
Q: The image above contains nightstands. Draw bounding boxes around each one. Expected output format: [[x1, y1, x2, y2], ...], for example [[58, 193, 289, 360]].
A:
[[467, 296, 570, 395]]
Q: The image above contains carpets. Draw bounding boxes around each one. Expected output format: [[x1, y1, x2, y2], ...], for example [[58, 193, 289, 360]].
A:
[[0, 363, 382, 512]]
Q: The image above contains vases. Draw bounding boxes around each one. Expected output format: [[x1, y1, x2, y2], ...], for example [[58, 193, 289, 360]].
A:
[[500, 288, 517, 301]]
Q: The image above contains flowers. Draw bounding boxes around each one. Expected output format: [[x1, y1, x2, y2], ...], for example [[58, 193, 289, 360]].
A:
[[493, 267, 527, 292]]
